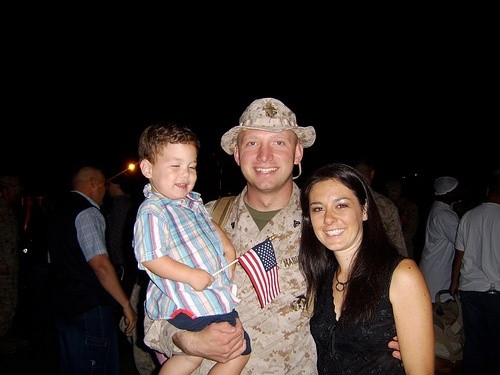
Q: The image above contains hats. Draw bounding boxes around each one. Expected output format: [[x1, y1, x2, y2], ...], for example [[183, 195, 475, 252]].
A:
[[220, 97, 317, 155], [433, 176, 458, 195]]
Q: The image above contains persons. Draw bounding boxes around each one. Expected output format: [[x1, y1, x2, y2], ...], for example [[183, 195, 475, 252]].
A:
[[132, 122, 253, 375], [0, 156, 500, 375], [143, 97, 404, 375], [297, 162, 435, 375]]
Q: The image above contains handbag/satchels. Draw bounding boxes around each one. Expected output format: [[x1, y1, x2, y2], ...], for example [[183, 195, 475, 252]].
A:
[[432, 289, 465, 364]]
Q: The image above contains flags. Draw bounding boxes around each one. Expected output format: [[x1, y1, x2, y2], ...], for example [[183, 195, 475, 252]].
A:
[[238, 239, 281, 310]]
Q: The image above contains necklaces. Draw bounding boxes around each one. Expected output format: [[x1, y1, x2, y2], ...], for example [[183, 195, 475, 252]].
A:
[[335, 267, 348, 291]]
